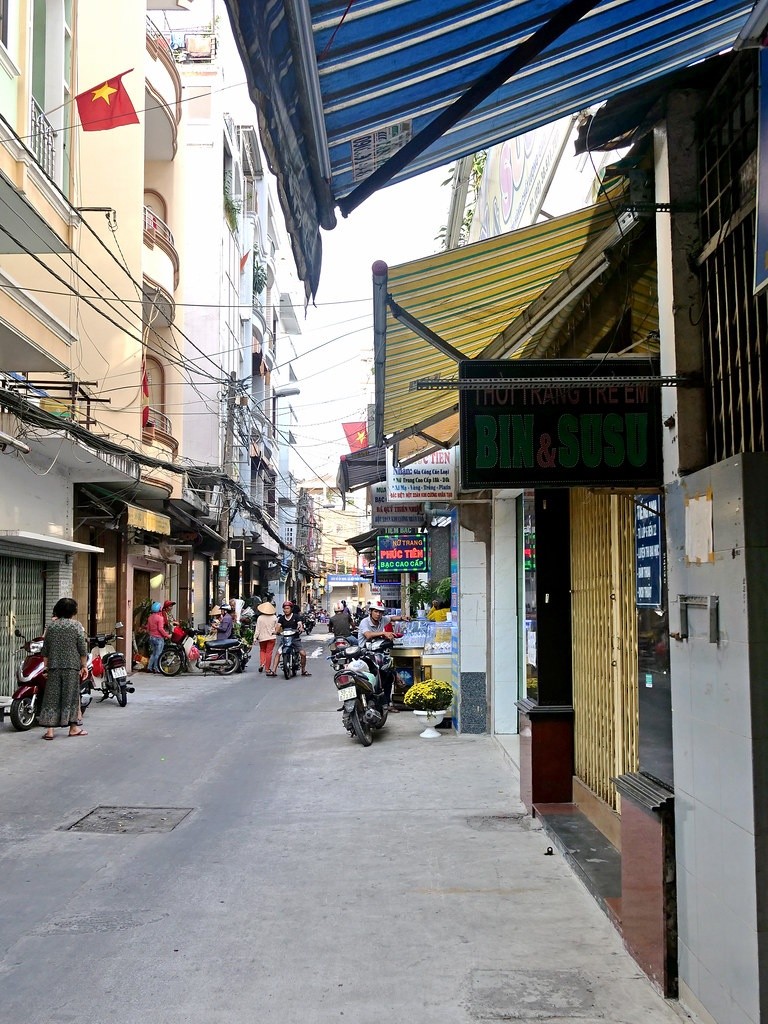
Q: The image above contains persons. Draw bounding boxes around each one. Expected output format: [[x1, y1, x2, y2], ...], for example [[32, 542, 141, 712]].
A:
[[209, 604, 232, 639], [303, 605, 330, 623], [253, 602, 278, 672], [266, 601, 312, 677], [358, 601, 412, 713], [356, 600, 371, 616], [341, 600, 350, 615], [328, 602, 358, 646], [426, 599, 451, 643], [146, 601, 176, 673], [39, 597, 88, 740]]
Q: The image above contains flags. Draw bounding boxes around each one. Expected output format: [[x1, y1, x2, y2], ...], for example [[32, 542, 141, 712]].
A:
[[76, 75, 139, 131], [142, 366, 149, 427], [342, 422, 368, 451]]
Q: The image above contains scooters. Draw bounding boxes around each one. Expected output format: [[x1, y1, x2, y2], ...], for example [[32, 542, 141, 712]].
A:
[[329, 641, 391, 746], [9, 629, 95, 731], [326, 624, 404, 687]]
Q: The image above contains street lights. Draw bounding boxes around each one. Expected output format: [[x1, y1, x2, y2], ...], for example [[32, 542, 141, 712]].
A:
[[291, 504, 334, 601], [217, 387, 301, 616]]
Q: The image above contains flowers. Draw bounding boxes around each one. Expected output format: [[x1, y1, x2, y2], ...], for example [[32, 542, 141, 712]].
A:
[[403, 679, 453, 710]]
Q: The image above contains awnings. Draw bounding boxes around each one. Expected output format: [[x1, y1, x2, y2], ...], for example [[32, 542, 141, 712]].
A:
[[373, 195, 643, 468], [336, 444, 386, 511], [226, 0, 762, 313]]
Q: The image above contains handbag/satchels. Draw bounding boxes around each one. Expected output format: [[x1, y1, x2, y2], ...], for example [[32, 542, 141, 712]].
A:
[[347, 658, 370, 672], [188, 646, 199, 662], [92, 655, 105, 677], [172, 627, 184, 643]]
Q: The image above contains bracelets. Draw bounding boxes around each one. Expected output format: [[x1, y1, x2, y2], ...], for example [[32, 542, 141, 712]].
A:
[[401, 617, 402, 621]]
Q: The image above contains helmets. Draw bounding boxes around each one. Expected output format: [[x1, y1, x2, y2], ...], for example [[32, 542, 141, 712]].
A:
[[334, 602, 344, 612], [220, 604, 231, 611], [283, 601, 294, 608], [369, 602, 385, 611]]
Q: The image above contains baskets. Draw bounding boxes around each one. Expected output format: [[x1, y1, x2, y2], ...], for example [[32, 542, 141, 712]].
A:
[[169, 636, 182, 648]]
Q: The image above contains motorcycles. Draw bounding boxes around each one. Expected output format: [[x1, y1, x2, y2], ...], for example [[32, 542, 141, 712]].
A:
[[272, 627, 303, 679], [80, 621, 135, 707], [157, 623, 243, 676], [297, 613, 314, 635]]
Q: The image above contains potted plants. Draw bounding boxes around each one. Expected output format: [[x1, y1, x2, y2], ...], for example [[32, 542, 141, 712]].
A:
[[403, 580, 434, 619]]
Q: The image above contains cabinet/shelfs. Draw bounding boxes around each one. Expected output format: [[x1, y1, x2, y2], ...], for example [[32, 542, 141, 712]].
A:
[[388, 620, 454, 686]]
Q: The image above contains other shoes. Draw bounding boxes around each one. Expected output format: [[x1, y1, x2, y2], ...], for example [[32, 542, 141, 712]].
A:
[[147, 667, 153, 673], [259, 667, 263, 672], [265, 669, 272, 672], [388, 706, 399, 713], [153, 669, 160, 674]]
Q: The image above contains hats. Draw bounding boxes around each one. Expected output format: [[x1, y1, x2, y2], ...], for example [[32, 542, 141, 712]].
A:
[[163, 601, 176, 607], [209, 605, 221, 615], [257, 602, 276, 614], [151, 602, 160, 612]]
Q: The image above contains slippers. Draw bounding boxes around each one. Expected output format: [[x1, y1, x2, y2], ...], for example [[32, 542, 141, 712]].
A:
[[42, 733, 53, 740], [301, 671, 312, 675], [266, 670, 277, 676], [68, 730, 88, 736]]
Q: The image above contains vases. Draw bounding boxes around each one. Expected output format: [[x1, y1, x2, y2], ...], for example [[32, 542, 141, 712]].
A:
[[413, 710, 446, 738]]
